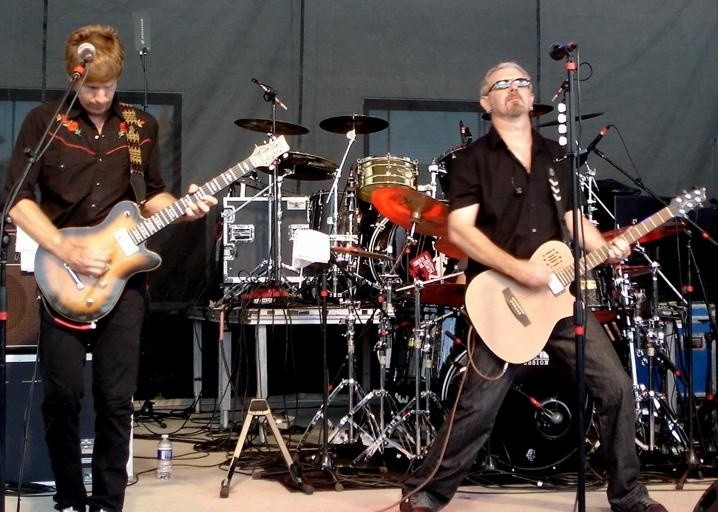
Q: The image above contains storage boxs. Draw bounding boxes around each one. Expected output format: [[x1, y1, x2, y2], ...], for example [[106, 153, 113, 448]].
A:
[[222, 197, 313, 284], [4, 342, 134, 491], [626, 305, 716, 400]]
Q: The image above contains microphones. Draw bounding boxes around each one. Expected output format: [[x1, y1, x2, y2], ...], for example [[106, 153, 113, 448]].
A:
[[549, 41, 576, 61], [71, 43, 96, 77], [576, 123, 612, 168]]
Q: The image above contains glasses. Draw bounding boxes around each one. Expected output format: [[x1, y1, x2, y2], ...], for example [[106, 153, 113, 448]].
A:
[[485, 78, 530, 96]]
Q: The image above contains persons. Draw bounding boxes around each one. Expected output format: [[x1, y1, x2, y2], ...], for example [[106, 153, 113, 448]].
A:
[[3, 25, 219, 512], [400, 61, 667, 512]]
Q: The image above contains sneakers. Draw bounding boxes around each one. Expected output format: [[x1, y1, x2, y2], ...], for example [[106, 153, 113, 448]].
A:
[[611, 496, 666, 512], [400, 493, 435, 511]]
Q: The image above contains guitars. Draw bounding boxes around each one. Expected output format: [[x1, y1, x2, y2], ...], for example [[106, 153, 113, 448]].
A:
[[34, 133, 290, 324], [465, 186, 708, 365]]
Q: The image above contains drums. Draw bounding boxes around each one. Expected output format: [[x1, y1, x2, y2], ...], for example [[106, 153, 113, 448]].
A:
[[615, 265, 657, 322], [435, 144, 471, 200], [370, 217, 450, 296], [306, 190, 360, 273], [355, 153, 418, 202], [440, 346, 594, 475]]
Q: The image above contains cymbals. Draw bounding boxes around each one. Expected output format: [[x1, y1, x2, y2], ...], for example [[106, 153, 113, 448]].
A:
[[403, 282, 466, 307], [539, 112, 604, 129], [331, 242, 394, 263], [319, 115, 389, 135], [371, 188, 451, 235], [600, 221, 685, 242], [479, 103, 553, 121], [257, 152, 340, 181], [234, 118, 310, 136]]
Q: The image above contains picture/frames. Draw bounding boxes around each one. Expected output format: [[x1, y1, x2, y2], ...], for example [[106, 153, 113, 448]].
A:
[[1, 89, 184, 202], [364, 99, 489, 185]]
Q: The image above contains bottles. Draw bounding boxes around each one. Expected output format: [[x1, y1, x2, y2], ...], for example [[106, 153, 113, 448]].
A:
[[157, 434, 173, 479]]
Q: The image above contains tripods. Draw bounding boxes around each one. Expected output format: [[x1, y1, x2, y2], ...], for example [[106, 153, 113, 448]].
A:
[[251, 77, 293, 309], [220, 399, 314, 498], [132, 54, 190, 428], [214, 134, 303, 308], [305, 228, 415, 462], [326, 131, 380, 305], [342, 270, 470, 475], [226, 166, 309, 306], [594, 150, 718, 490], [294, 274, 383, 465], [356, 307, 465, 478], [576, 171, 703, 479], [253, 264, 388, 493]]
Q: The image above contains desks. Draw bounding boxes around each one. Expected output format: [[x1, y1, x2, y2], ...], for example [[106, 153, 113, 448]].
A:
[[189, 305, 383, 430]]
